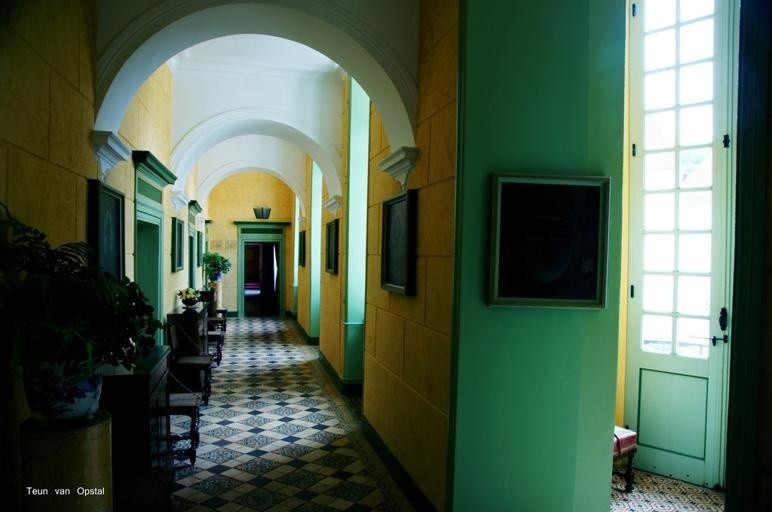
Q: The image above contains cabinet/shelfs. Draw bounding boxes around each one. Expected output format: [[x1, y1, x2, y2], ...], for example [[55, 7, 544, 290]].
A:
[[101, 343, 173, 511]]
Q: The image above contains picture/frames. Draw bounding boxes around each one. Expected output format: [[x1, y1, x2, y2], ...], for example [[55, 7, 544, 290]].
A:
[[380, 189, 417, 300], [491, 173, 610, 308], [172, 217, 185, 272], [296, 228, 306, 268], [86, 181, 125, 282], [324, 218, 339, 274]]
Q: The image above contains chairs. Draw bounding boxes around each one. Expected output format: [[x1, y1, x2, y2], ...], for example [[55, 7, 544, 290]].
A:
[[164, 287, 228, 466]]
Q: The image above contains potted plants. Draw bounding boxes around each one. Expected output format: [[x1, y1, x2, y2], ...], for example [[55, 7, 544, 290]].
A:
[[0, 201, 171, 427], [201, 249, 233, 283]]
